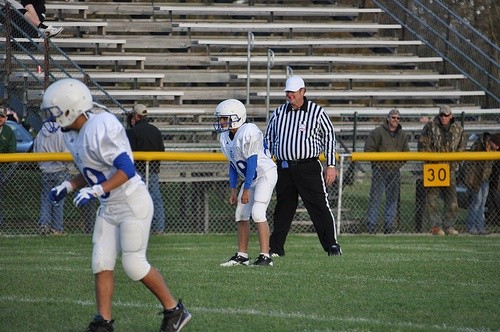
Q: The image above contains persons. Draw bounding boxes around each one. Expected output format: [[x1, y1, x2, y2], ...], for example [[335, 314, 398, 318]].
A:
[[364, 109, 410, 233], [458, 132, 500, 235], [126, 112, 135, 127], [122, 102, 165, 233], [39, 79, 192, 332], [263, 75, 342, 257], [417, 105, 467, 234], [0, 106, 20, 186], [212, 98, 278, 267], [32, 117, 73, 235], [0, 0, 64, 50]]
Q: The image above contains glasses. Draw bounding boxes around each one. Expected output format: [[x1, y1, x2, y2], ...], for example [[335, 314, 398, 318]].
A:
[[391, 116, 400, 120], [440, 114, 450, 117]]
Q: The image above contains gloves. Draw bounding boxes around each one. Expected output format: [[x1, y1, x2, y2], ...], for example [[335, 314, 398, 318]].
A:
[[72, 184, 105, 209], [47, 181, 73, 207]]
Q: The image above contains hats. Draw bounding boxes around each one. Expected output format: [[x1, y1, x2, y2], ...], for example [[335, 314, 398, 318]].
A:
[[283, 76, 305, 92], [133, 104, 148, 116], [388, 109, 400, 117], [490, 133, 500, 147], [439, 105, 451, 115], [0, 106, 7, 118]]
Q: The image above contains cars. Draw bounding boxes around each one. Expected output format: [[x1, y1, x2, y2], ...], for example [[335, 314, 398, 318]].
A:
[[5, 121, 38, 170]]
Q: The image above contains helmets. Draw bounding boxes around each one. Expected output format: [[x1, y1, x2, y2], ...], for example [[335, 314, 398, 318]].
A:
[[40, 78, 93, 127], [216, 99, 246, 129]]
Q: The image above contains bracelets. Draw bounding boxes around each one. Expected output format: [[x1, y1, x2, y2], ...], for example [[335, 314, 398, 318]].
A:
[[329, 165, 335, 168]]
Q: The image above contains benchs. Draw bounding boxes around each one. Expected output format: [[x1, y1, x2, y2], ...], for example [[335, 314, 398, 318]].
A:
[[0, 0, 500, 226]]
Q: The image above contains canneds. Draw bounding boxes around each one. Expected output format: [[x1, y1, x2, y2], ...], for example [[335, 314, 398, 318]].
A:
[[37, 64, 41, 73]]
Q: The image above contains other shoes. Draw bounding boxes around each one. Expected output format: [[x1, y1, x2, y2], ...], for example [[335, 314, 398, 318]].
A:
[[367, 228, 377, 234], [431, 226, 445, 235], [446, 227, 459, 235], [384, 228, 402, 234], [467, 227, 488, 235], [41, 225, 66, 236], [328, 245, 343, 256]]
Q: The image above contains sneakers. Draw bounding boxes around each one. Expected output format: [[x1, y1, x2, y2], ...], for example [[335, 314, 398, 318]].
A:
[[220, 252, 249, 267], [249, 254, 274, 267], [158, 298, 192, 332], [38, 24, 64, 38], [85, 315, 115, 332], [270, 253, 281, 258]]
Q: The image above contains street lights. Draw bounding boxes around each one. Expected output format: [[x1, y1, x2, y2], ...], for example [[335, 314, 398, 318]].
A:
[[23, 71, 28, 101]]
